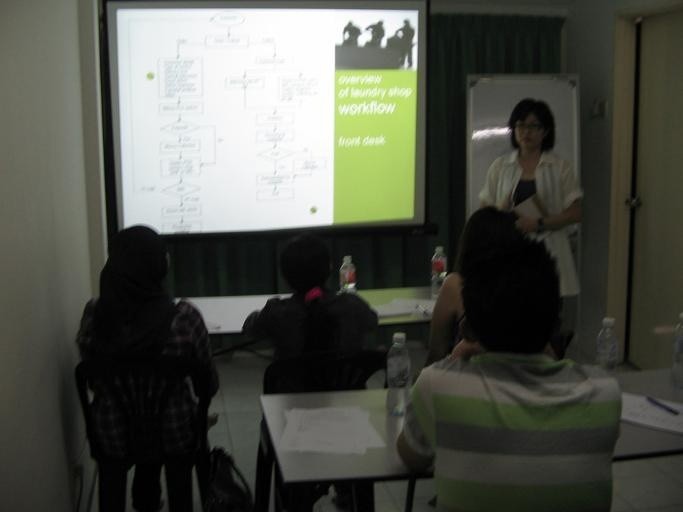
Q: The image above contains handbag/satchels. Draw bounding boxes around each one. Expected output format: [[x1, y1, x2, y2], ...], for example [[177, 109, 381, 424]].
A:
[[199, 446, 253, 512]]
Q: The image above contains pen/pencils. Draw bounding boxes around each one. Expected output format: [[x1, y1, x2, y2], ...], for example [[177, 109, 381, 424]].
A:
[[646, 395, 679, 415]]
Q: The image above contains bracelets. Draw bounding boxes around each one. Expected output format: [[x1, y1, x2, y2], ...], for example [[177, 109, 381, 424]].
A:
[[537, 218, 544, 234]]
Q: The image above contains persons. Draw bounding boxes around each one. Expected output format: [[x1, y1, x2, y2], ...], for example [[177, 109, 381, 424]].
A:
[[395, 238, 623, 512], [424, 205, 521, 365], [75, 224, 218, 512], [240, 233, 381, 511], [476, 97, 585, 361]]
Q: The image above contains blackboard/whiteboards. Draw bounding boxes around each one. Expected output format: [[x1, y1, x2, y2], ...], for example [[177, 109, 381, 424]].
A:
[[466, 72, 581, 247]]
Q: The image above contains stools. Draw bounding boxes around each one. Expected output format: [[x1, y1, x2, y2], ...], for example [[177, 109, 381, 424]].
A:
[[253, 382, 417, 511]]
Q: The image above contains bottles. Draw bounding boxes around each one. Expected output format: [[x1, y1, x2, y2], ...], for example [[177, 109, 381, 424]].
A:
[[670, 313, 683, 396], [429, 246, 448, 298], [595, 315, 620, 379], [386, 332, 413, 416], [338, 255, 357, 295]]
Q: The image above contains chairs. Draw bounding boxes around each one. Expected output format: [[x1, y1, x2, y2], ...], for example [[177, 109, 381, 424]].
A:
[[73, 343, 219, 510]]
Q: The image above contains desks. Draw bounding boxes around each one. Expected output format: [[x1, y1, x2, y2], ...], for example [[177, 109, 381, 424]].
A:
[[169, 286, 437, 358], [260, 370, 682, 512]]
